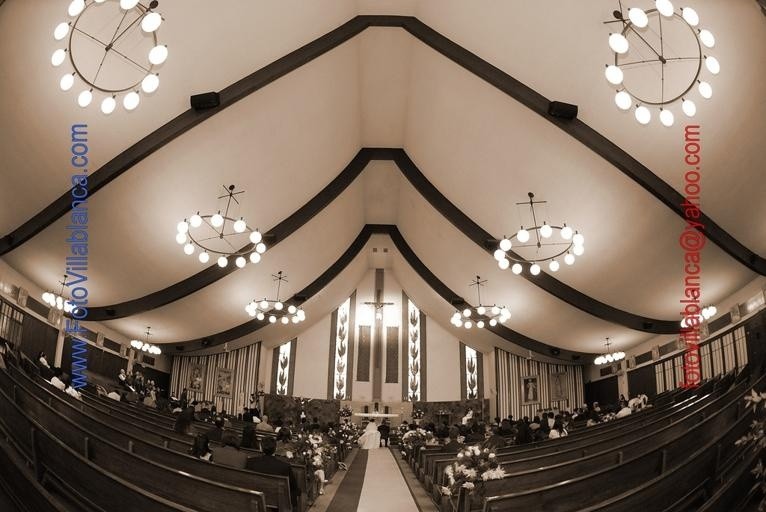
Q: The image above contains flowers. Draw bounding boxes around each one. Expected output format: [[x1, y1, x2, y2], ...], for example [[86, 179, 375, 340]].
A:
[[339, 404, 354, 416], [341, 421, 362, 450]]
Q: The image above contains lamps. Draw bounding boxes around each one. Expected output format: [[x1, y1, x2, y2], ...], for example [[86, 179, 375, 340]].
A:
[[450, 275, 513, 329], [680, 290, 717, 328], [604, 0, 723, 128], [176, 183, 266, 268], [594, 337, 626, 366], [49, 0, 169, 113], [494, 192, 585, 276], [42, 274, 79, 316], [130, 326, 162, 356], [244, 271, 306, 325]]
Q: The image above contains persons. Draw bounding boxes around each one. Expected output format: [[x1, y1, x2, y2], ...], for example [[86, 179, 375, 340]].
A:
[[38, 351, 651, 509]]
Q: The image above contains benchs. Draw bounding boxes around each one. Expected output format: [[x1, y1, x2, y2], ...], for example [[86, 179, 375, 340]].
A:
[[1, 344, 340, 512], [400, 361, 764, 510]]
